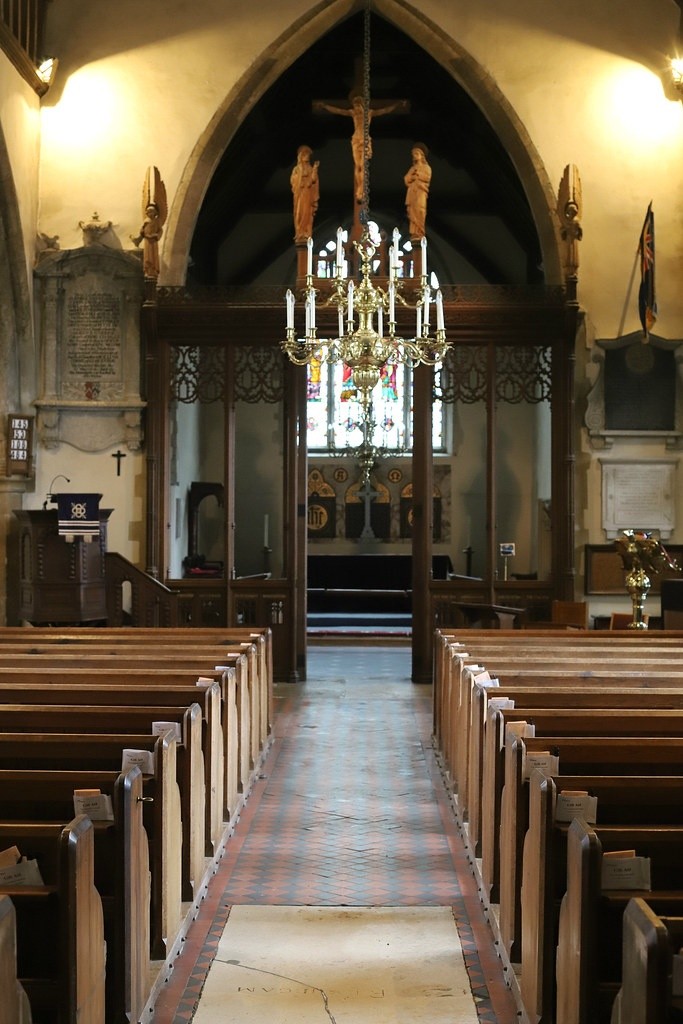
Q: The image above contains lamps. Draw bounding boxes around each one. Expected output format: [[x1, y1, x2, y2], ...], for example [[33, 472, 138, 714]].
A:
[[277, 0, 455, 487]]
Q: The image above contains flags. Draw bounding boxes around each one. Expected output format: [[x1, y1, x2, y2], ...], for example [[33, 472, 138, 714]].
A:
[[638, 206, 658, 339]]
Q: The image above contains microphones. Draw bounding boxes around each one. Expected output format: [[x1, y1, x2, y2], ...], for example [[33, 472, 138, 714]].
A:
[[49, 474, 70, 495]]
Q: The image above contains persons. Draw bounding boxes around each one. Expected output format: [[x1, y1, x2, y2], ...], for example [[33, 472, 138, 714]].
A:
[[132, 203, 162, 278], [404, 148, 431, 238], [316, 96, 403, 203], [290, 149, 320, 240], [559, 204, 583, 278]]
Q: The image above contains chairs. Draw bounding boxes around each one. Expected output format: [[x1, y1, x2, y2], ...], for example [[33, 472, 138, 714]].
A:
[[609, 612, 650, 630], [549, 599, 591, 630]]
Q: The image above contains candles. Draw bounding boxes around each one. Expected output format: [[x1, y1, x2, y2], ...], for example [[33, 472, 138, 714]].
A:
[[263, 514, 270, 548], [465, 514, 471, 548]]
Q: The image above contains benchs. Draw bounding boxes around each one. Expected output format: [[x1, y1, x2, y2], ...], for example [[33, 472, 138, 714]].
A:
[[431, 627, 683, 1024], [0, 623, 275, 1024]]
